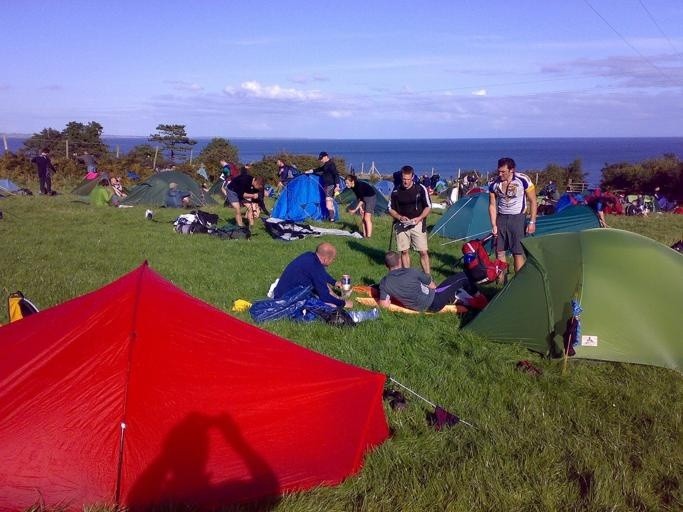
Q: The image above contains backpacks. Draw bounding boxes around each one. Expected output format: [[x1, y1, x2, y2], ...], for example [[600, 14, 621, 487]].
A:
[[229, 162, 237, 177], [462, 235, 508, 286], [393, 171, 420, 196]]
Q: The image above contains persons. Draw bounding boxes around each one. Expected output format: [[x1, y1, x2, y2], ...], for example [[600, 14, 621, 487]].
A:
[[377, 250, 488, 313], [273, 242, 354, 310], [488, 158, 537, 286], [386, 165, 433, 289], [28, 147, 682, 242]]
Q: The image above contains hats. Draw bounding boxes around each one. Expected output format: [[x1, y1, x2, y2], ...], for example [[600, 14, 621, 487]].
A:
[[318, 152, 328, 160], [169, 183, 179, 188]]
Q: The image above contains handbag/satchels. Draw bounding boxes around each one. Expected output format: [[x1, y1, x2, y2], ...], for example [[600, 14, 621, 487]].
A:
[[219, 228, 251, 240], [45, 167, 53, 178]]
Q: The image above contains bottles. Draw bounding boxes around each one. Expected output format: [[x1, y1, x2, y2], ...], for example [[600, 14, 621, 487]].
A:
[[342, 274, 351, 290]]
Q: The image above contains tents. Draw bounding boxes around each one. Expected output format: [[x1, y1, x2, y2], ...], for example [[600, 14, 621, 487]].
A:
[[456, 228, 683, 373], [430, 191, 517, 258], [0, 256, 391, 512]]
